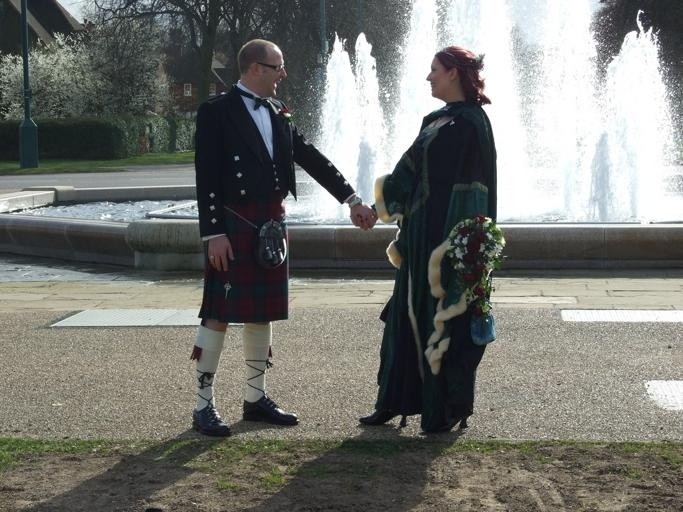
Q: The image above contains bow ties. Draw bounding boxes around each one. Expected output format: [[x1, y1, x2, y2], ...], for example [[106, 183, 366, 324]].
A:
[[254, 98, 268, 110]]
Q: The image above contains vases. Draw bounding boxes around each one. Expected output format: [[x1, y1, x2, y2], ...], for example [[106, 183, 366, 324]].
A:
[[469, 311, 496, 347]]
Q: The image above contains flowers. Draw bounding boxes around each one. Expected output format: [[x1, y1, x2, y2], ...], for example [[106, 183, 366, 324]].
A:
[[447, 215, 504, 315]]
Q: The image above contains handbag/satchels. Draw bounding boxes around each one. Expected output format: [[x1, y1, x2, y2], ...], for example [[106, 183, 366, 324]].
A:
[[254, 219, 287, 269]]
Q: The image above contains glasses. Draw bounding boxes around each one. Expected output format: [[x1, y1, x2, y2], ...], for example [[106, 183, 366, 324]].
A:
[[257, 62, 284, 71]]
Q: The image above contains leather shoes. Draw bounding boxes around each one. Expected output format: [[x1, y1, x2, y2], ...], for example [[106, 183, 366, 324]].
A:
[[243, 396, 299, 424], [192, 406, 231, 436]]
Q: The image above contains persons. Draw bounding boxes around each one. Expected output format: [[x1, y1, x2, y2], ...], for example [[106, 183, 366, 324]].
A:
[[354, 46, 497, 432], [189, 37, 375, 439]]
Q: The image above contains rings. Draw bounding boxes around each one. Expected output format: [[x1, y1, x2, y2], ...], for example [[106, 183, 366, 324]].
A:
[[210, 255, 214, 260]]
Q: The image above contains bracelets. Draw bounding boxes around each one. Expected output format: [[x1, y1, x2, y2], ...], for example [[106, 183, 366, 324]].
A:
[[348, 199, 360, 210]]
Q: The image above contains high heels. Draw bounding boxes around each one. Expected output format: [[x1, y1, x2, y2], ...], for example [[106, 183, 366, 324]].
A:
[[360, 408, 406, 428], [423, 409, 472, 432]]
